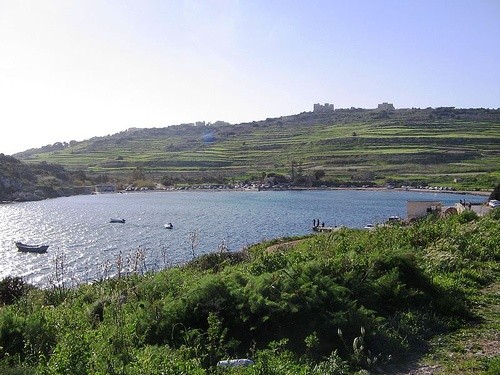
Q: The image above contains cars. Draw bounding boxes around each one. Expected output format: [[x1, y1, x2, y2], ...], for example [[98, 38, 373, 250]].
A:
[[489, 200, 500, 208]]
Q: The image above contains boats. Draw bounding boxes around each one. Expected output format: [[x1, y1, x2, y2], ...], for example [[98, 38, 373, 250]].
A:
[[165, 223, 174, 230], [110, 217, 124, 225], [14, 242, 49, 253]]
